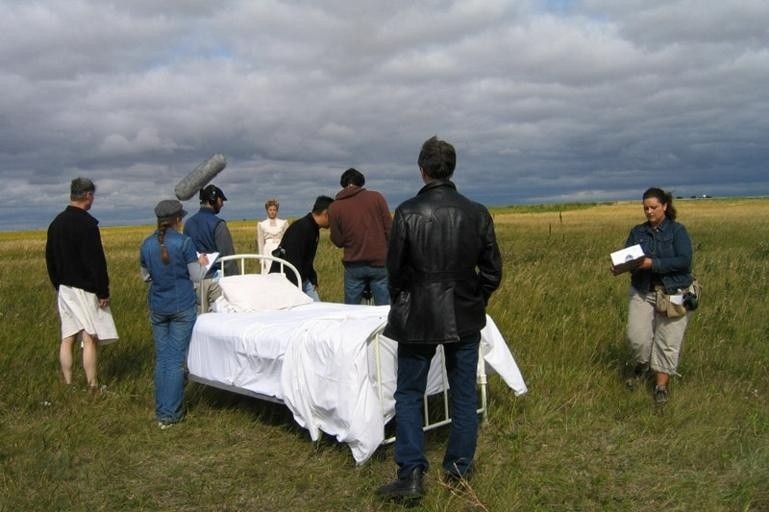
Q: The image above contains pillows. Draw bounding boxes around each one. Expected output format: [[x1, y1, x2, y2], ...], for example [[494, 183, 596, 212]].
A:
[[218, 273, 314, 311]]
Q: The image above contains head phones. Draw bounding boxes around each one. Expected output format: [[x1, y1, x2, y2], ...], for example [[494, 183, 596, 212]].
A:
[[208, 185, 217, 205]]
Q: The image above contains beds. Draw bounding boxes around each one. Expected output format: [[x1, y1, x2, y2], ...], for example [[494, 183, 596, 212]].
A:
[[187, 254, 529, 466]]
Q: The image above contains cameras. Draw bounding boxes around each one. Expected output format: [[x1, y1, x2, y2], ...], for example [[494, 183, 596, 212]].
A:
[[272, 245, 287, 257]]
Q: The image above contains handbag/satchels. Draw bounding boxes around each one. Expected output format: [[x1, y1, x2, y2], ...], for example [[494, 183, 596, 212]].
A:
[[655, 277, 701, 319]]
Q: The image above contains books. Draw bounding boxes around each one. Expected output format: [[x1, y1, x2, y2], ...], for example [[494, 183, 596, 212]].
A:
[[193, 249, 220, 279]]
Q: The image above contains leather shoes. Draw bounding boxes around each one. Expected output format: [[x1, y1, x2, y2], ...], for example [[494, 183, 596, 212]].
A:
[[374, 466, 425, 505], [445, 473, 471, 495]]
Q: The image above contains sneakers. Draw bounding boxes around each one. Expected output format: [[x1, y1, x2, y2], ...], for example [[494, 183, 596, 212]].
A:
[[626, 364, 650, 392], [653, 385, 670, 406]]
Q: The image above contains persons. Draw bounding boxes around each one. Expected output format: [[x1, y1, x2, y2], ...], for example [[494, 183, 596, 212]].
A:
[[44, 177, 117, 393], [609, 187, 696, 401], [375, 135, 504, 503], [183, 185, 239, 276], [255, 199, 291, 275], [139, 200, 210, 430], [328, 168, 397, 306], [270, 195, 336, 303]]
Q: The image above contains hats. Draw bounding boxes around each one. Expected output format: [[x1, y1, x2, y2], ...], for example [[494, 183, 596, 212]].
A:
[[203, 185, 227, 201], [153, 199, 189, 216]]
